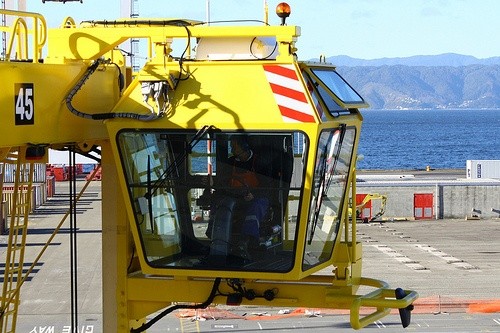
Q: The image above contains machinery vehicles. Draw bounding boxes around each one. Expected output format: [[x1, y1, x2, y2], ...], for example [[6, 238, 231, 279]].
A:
[[1, 0, 419, 333]]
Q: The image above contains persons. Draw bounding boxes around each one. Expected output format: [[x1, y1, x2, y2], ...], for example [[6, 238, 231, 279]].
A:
[[202, 134, 269, 258]]
